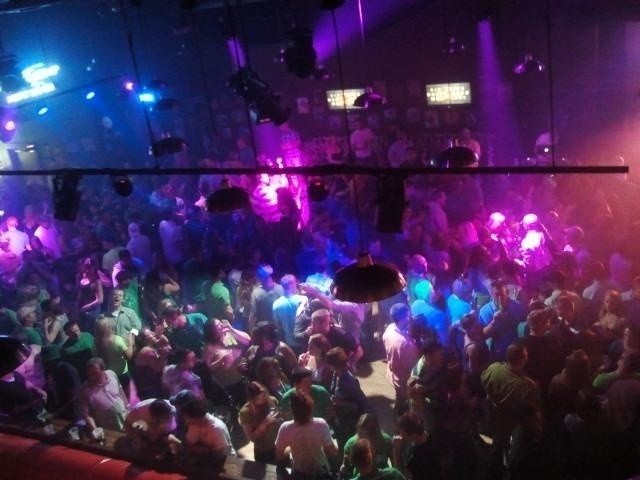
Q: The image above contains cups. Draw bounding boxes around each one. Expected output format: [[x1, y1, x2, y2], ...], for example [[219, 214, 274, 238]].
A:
[[92, 428, 105, 442], [43, 424, 54, 433], [70, 427, 80, 440]]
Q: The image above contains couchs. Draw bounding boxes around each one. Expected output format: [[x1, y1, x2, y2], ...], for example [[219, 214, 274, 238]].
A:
[[0, 431, 196, 479]]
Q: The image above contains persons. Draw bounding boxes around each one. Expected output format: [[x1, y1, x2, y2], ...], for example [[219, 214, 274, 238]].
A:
[[0, 61, 640, 480]]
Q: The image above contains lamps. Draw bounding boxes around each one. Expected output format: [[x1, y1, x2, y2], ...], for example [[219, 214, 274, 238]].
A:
[[118, 0, 559, 304], [0, 168, 628, 232]]
